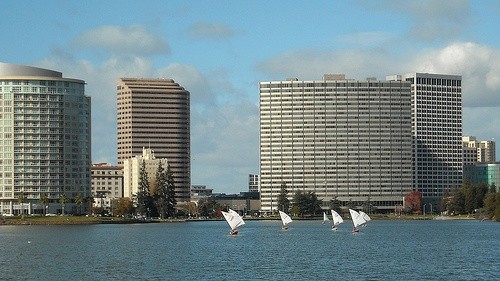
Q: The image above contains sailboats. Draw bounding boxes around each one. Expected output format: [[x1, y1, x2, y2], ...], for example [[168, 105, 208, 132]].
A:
[[349, 209, 366, 233], [222, 209, 245, 235], [359, 211, 371, 227], [331, 209, 343, 231], [280, 211, 292, 230], [321, 212, 330, 224]]
